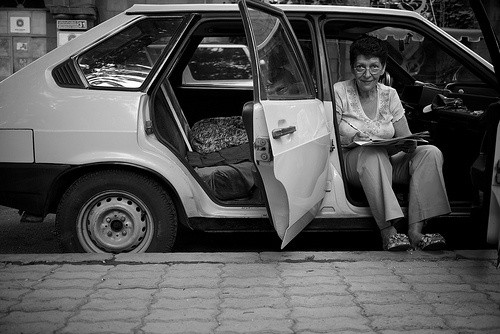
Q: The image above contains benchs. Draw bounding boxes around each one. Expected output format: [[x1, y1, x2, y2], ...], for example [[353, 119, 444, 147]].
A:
[[145, 61, 257, 199]]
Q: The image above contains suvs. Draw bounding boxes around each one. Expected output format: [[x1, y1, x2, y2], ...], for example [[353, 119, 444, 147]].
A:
[[0, 0, 500, 254]]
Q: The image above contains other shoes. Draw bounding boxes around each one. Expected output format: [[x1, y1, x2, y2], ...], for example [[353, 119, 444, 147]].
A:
[[411, 233, 447, 251], [382, 233, 411, 252]]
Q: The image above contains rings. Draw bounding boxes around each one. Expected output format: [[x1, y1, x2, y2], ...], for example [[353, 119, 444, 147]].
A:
[[408, 149, 410, 152]]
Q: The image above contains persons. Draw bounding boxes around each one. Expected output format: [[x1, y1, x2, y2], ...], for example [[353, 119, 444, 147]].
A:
[[333, 36, 452, 251], [263, 67, 300, 96]]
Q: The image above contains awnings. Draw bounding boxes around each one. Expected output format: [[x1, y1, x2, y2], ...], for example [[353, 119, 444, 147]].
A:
[[366, 27, 485, 51]]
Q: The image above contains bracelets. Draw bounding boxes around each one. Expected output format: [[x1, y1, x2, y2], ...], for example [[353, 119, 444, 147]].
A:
[[352, 131, 359, 141]]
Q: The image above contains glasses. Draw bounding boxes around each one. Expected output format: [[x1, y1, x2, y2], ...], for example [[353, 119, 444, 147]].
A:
[[351, 61, 384, 75]]
